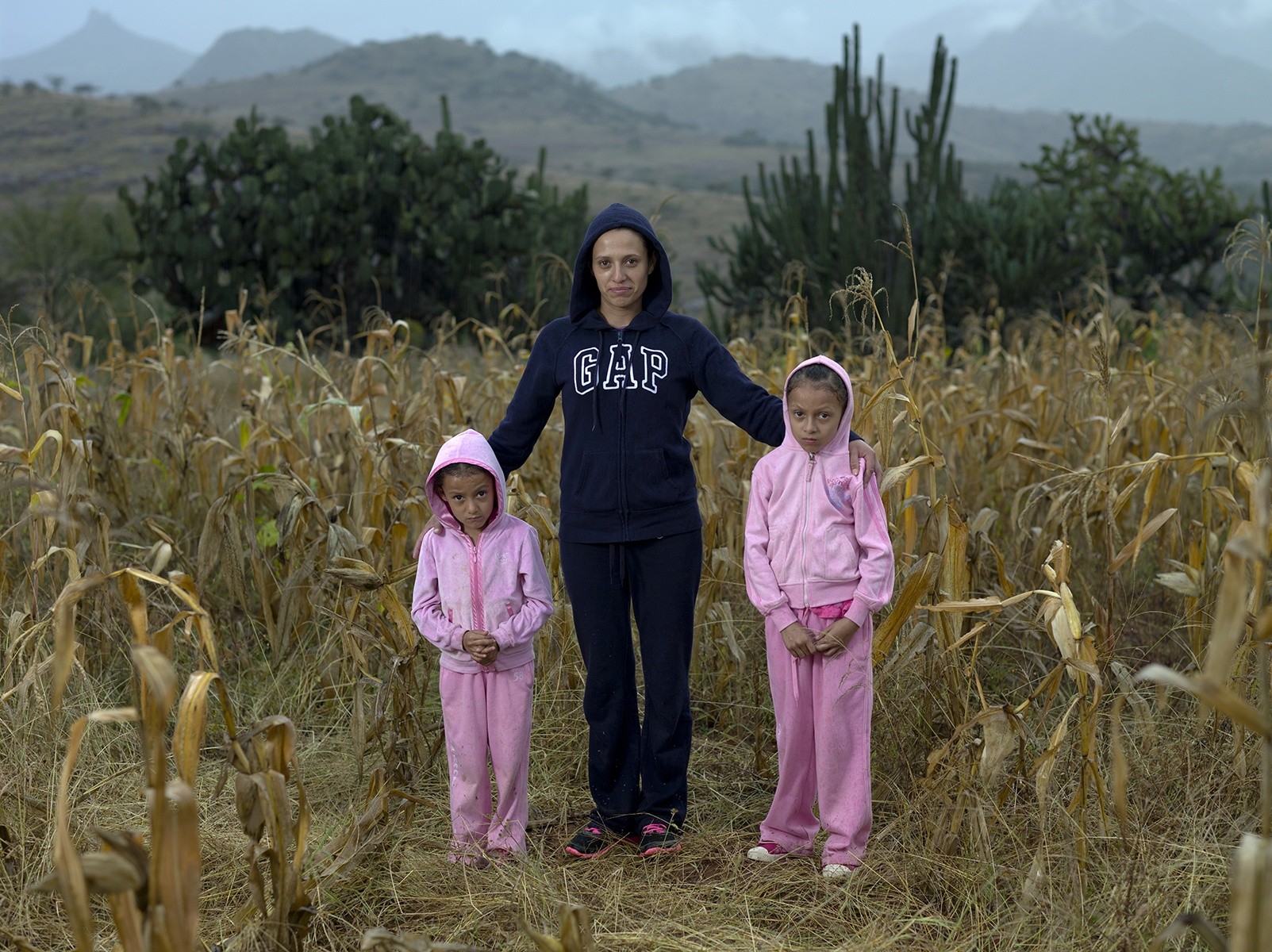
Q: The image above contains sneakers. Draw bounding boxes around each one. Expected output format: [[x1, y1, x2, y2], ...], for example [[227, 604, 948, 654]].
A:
[[642, 822, 681, 856], [823, 863, 859, 879], [565, 821, 642, 856], [747, 842, 793, 862]]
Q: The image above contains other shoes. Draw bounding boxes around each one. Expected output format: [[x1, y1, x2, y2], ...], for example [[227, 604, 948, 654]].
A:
[[493, 849, 517, 873], [447, 854, 486, 868]]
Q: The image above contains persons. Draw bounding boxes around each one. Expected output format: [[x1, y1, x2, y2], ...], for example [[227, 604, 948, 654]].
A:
[[411, 430, 553, 867], [743, 355, 895, 881], [413, 202, 883, 858]]
